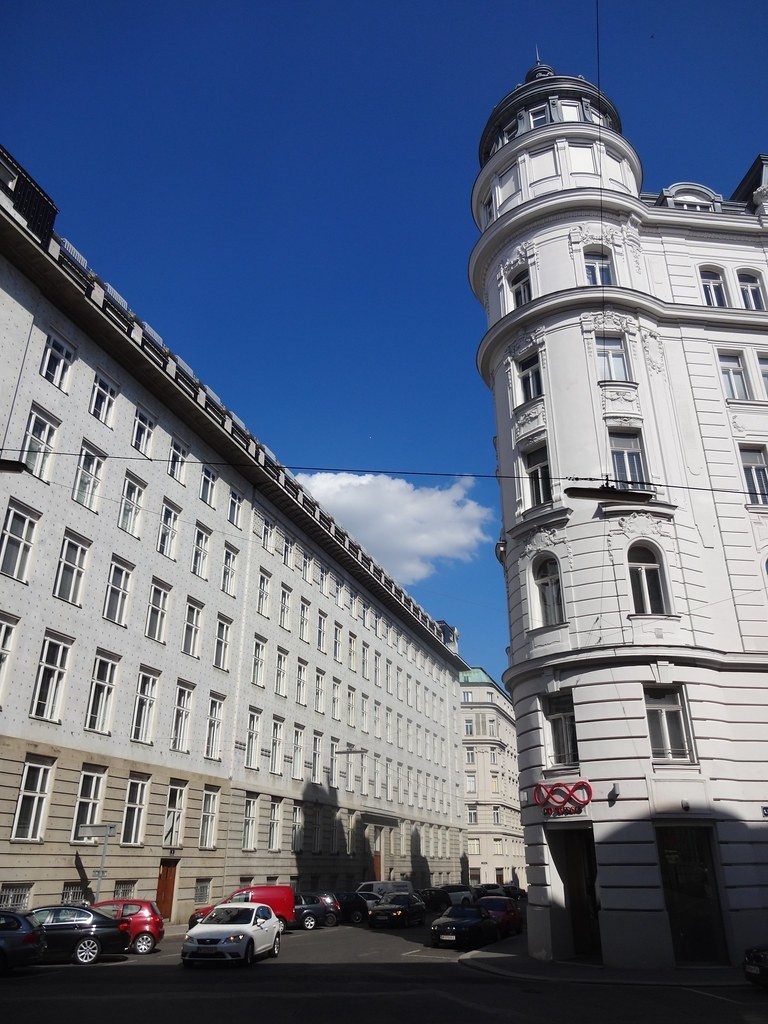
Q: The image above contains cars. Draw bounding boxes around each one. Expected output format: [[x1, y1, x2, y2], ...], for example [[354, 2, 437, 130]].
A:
[[477, 895, 523, 935], [0, 908, 48, 980], [12, 904, 132, 965], [294, 892, 328, 931], [66, 899, 165, 956], [365, 890, 426, 930], [309, 890, 344, 928], [431, 903, 496, 954], [180, 901, 281, 966], [334, 879, 526, 911]]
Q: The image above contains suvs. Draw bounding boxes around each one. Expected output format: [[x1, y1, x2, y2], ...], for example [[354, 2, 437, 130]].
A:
[[188, 884, 296, 935]]
